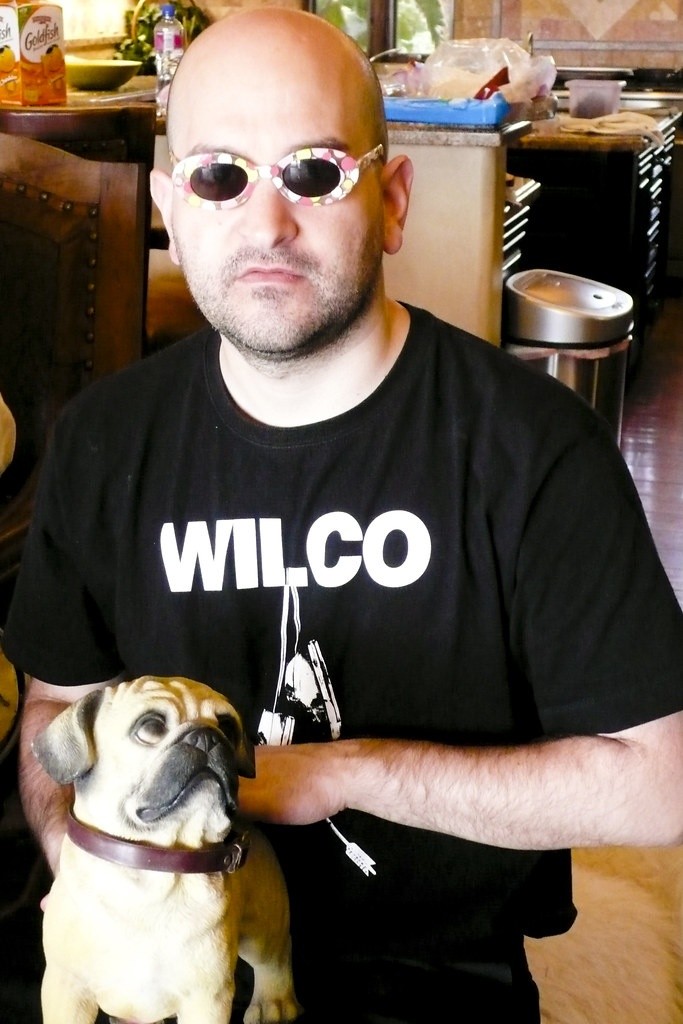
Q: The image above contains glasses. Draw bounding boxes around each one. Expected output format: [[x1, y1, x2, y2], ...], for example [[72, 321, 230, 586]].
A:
[[169, 144, 386, 211]]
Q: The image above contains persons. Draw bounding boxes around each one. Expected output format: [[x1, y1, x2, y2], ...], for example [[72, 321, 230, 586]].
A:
[[0, 0, 683, 1024]]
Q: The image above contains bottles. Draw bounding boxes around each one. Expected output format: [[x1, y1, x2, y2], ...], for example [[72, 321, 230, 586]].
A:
[[153, 5, 185, 100]]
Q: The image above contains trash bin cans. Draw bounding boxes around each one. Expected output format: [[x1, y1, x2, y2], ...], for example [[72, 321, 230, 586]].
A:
[[501, 263, 634, 452]]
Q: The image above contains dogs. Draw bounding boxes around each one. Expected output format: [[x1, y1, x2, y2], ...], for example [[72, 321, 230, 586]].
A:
[[30, 674, 305, 1024]]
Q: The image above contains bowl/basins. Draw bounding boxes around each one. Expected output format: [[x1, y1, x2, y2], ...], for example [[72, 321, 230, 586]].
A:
[[565, 79, 627, 119], [66, 60, 143, 89]]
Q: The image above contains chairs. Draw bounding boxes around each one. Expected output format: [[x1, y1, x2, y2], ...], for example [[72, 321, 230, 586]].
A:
[[1, 106, 157, 593]]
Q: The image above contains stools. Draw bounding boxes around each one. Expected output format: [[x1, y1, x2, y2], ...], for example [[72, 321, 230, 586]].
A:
[[505, 337, 629, 453]]
